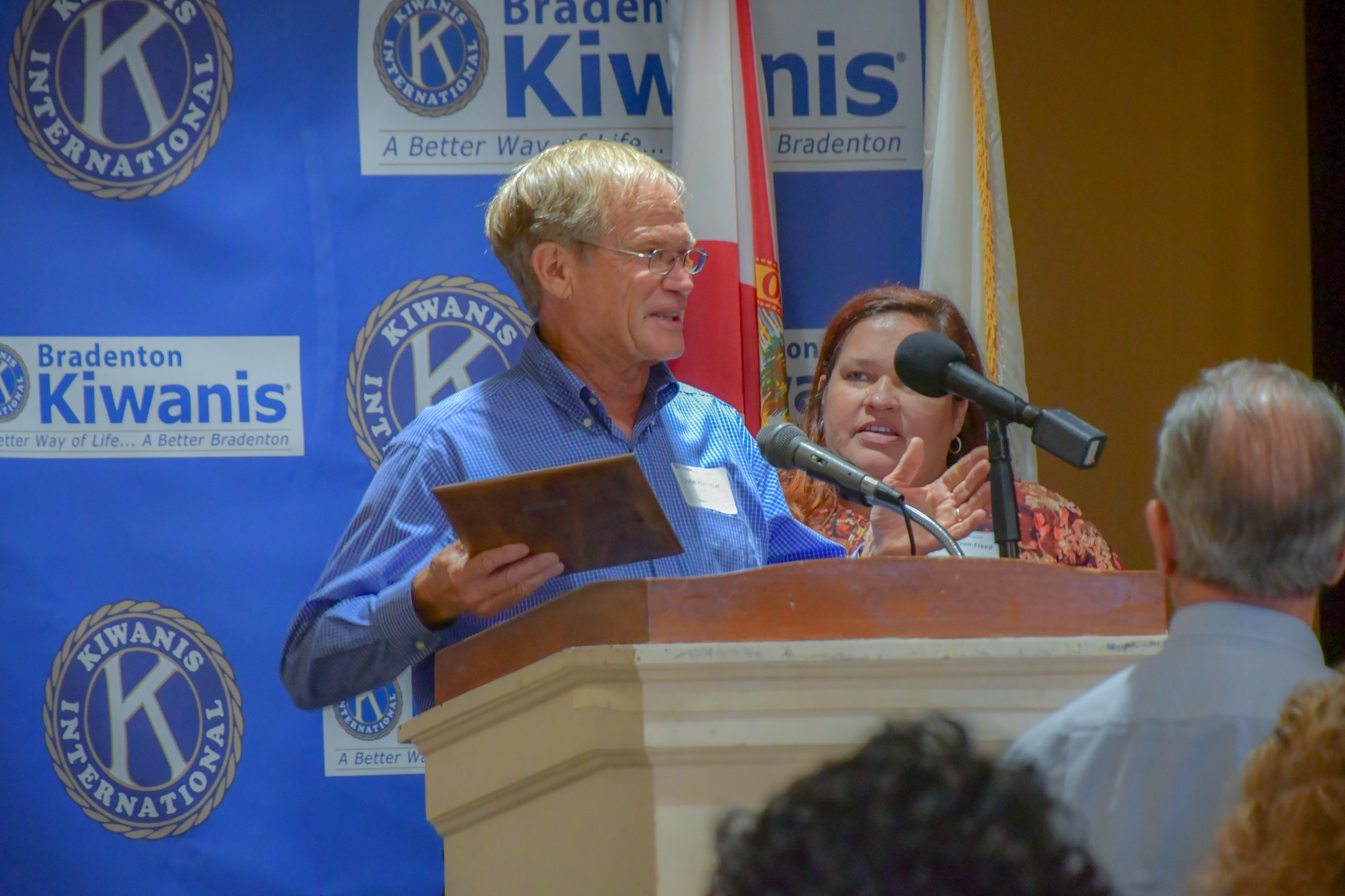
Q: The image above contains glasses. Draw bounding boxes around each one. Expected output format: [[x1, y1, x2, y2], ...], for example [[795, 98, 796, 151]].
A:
[[570, 237, 710, 276]]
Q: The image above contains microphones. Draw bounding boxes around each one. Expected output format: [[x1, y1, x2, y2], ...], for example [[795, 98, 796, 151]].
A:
[[757, 421, 906, 504], [894, 331, 1106, 469]]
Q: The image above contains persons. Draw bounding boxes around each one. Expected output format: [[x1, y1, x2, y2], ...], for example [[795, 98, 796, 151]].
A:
[[1189, 677, 1345, 895], [978, 358, 1343, 896], [704, 709, 1118, 896], [281, 139, 992, 711], [776, 277, 1124, 574]]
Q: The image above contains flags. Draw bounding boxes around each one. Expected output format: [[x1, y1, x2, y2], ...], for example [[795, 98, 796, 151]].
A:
[[911, 0, 1043, 494], [658, 0, 794, 435]]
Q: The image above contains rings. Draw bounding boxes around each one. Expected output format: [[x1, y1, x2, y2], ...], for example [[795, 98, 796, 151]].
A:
[[954, 508, 964, 523]]
